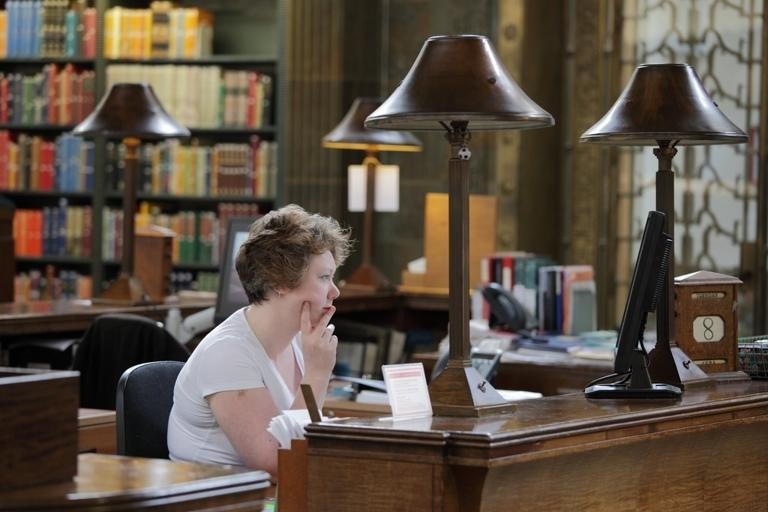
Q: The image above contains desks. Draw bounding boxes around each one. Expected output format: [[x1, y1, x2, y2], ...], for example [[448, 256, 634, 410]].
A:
[[0, 454, 272, 512], [276, 353, 768, 512], [0, 283, 399, 385], [75, 407, 119, 456]]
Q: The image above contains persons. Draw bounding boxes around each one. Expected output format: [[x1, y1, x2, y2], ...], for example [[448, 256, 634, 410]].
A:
[[167, 204, 354, 512]]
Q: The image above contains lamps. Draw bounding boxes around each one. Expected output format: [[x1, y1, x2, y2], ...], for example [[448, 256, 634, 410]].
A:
[[578, 61, 753, 389], [320, 97, 425, 293], [71, 81, 192, 308], [363, 34, 556, 418]]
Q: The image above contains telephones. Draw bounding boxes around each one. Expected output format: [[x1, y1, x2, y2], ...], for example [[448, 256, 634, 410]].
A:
[[482, 282, 548, 343]]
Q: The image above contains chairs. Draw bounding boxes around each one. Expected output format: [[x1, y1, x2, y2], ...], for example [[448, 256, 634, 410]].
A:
[[117, 360, 188, 460], [70, 313, 191, 412]]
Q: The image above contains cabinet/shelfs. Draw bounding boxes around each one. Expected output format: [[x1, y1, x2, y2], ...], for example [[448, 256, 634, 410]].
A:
[[0, 0, 288, 302]]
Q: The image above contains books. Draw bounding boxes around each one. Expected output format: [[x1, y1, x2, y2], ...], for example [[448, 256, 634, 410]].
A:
[[483, 251, 597, 334], [1, 62, 96, 126], [104, 0, 214, 58], [136, 202, 167, 237], [105, 135, 278, 198], [12, 264, 94, 304], [0, 0, 96, 59], [0, 129, 96, 193], [12, 198, 125, 261], [105, 62, 273, 130]]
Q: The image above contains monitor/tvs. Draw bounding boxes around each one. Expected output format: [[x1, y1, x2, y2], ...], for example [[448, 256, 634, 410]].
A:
[[584, 210, 684, 399]]
